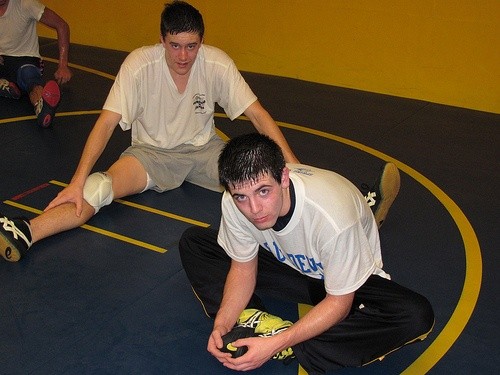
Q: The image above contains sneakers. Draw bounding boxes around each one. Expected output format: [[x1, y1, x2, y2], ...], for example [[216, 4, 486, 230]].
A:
[[0, 79, 21, 101], [360, 162, 401, 230], [231, 320, 299, 364], [218, 308, 284, 352], [36, 80, 60, 127], [0, 215, 32, 262]]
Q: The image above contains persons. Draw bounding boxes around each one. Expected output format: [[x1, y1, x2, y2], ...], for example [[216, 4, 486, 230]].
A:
[[173, 129, 437, 375], [0, 1, 404, 266], [0, 0, 75, 127]]
